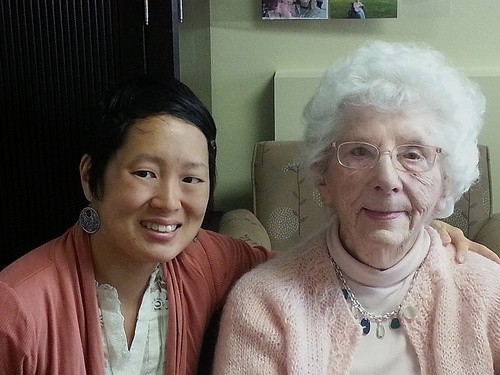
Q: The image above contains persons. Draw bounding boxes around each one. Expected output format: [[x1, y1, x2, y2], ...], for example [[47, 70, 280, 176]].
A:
[[0, 74, 500, 375], [212, 42, 500, 375], [291, 0, 366, 19]]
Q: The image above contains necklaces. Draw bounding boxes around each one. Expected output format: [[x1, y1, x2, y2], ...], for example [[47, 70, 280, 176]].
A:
[[97, 275, 163, 375], [326, 251, 418, 339]]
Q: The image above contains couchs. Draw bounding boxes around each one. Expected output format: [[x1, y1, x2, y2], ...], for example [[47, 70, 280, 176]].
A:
[[216, 139, 500, 262]]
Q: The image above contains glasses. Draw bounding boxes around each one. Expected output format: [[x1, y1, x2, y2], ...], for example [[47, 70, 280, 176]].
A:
[[331, 142, 443, 174]]
